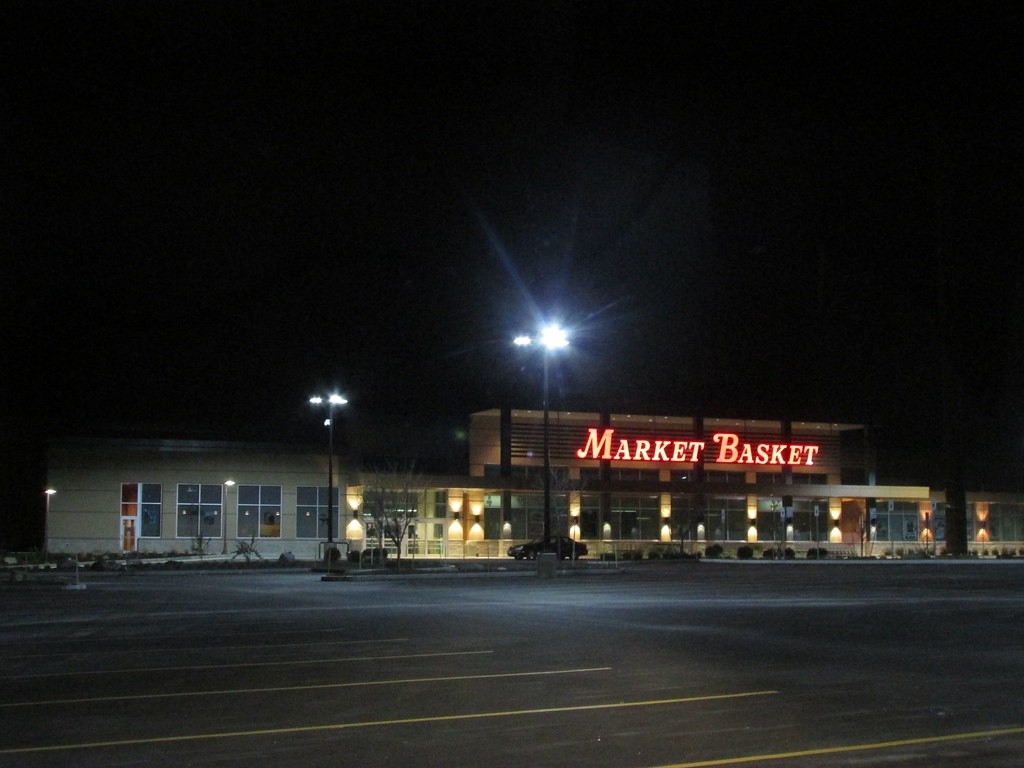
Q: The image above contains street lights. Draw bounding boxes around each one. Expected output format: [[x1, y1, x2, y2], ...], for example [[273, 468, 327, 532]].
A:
[[513, 324, 570, 541], [310, 382, 348, 561], [222, 478, 235, 553]]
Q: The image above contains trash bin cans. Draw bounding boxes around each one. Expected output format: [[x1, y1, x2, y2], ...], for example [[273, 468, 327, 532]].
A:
[[537, 553, 557, 579]]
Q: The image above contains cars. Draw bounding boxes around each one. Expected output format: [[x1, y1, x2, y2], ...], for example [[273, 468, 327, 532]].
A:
[[508, 535, 588, 561]]
